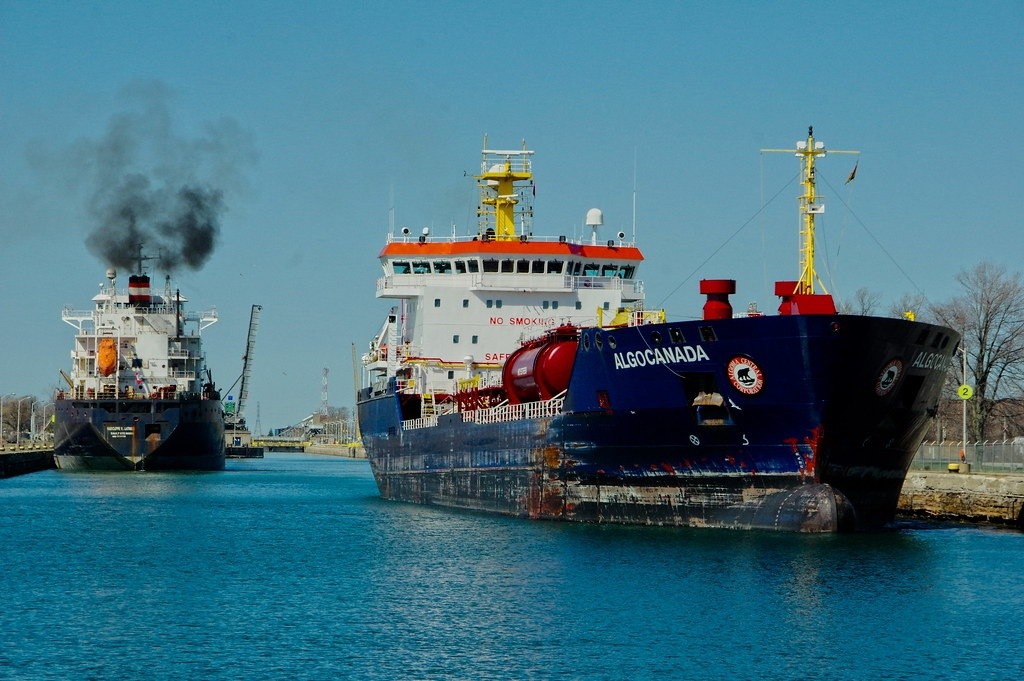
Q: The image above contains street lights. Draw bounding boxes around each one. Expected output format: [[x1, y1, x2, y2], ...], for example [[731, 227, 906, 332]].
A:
[[31, 400, 44, 441], [43, 403, 55, 441], [17, 397, 31, 443], [1, 392, 16, 443]]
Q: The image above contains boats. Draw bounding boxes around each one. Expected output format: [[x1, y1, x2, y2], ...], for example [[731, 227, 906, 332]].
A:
[[355, 126, 961, 534], [54, 242, 226, 472]]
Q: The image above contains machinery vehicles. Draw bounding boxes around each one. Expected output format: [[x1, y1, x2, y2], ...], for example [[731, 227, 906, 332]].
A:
[[348, 342, 364, 447]]
